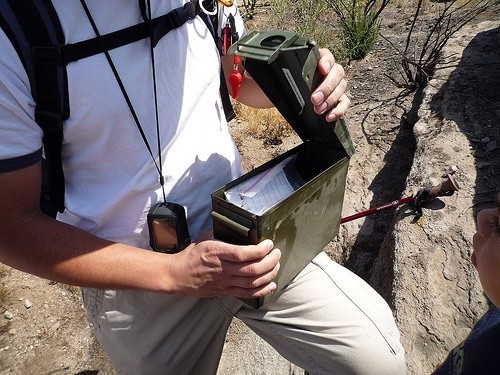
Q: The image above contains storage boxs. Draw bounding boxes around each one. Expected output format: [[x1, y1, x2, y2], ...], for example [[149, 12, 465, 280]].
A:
[[210, 30, 355, 309]]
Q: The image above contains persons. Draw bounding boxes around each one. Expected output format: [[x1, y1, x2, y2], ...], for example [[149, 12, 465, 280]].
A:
[[0, 0, 407, 375], [430, 207, 500, 375]]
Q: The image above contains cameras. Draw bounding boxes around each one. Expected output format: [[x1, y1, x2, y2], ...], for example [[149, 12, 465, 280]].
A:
[[147, 202, 191, 254]]
